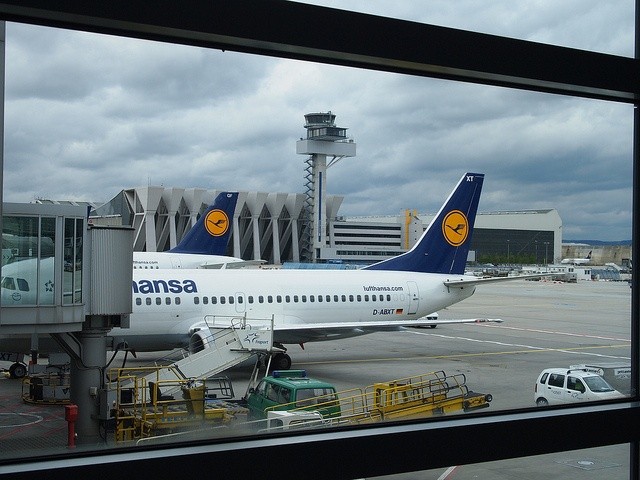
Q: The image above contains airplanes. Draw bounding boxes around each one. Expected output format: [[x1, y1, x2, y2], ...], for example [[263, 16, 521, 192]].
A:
[[0, 173, 556, 379], [0, 192, 267, 277]]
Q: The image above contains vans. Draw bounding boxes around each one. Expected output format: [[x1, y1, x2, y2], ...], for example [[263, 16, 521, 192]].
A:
[[246, 370, 340, 431]]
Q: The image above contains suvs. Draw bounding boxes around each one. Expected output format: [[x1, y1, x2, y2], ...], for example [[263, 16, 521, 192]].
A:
[[535, 368, 626, 407]]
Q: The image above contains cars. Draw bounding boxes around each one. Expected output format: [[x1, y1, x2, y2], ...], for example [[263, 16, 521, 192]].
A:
[[404, 312, 438, 328], [474, 263, 498, 275]]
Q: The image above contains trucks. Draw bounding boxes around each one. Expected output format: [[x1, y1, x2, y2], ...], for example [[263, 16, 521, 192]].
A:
[[267, 410, 324, 432]]
